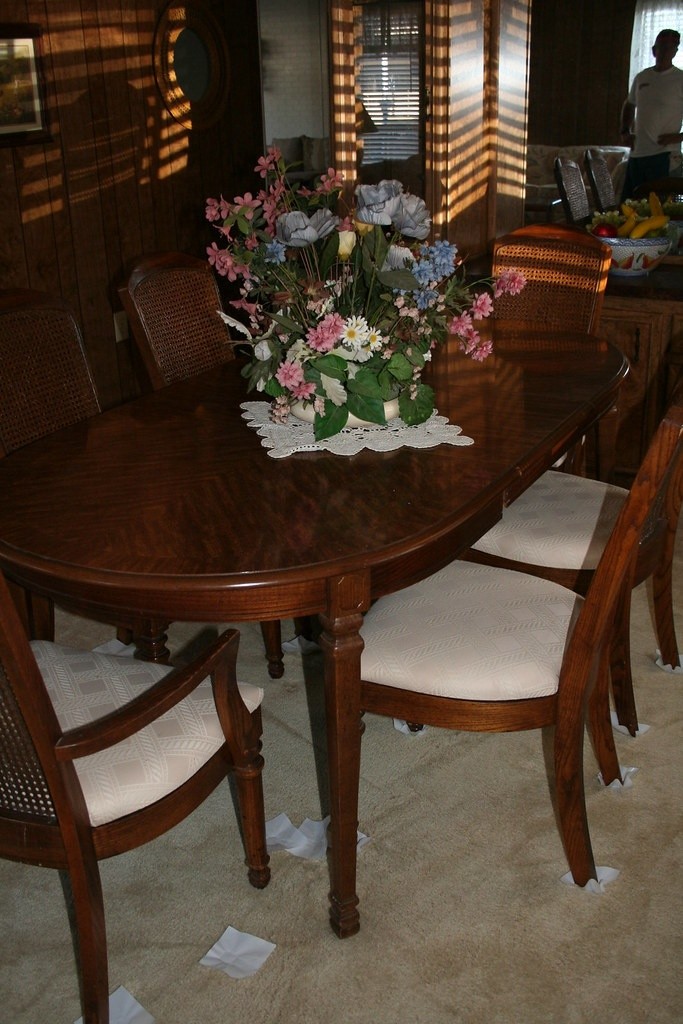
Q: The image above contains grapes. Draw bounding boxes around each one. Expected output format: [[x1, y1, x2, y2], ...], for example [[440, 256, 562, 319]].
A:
[[586, 199, 683, 232]]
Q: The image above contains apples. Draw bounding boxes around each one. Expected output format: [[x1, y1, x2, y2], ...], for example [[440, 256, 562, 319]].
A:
[[591, 223, 618, 237]]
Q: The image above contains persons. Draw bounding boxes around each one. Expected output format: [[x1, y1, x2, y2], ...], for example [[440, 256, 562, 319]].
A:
[[619, 29, 683, 204]]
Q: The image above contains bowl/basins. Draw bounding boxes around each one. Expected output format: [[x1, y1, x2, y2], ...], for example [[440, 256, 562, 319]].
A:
[[587, 221, 672, 277]]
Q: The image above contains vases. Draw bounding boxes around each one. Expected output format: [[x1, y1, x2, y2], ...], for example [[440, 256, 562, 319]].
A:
[[290, 397, 401, 428]]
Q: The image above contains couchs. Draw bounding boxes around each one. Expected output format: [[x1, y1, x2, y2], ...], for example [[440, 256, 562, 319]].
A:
[[525, 143, 630, 224]]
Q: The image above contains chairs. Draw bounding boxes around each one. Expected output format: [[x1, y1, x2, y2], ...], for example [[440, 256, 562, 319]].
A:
[[463, 364, 683, 738], [0, 573, 270, 1024], [554, 156, 591, 225], [0, 289, 103, 460], [361, 405, 683, 888], [117, 251, 237, 391], [584, 149, 617, 213], [0, 319, 631, 938], [491, 223, 612, 474]]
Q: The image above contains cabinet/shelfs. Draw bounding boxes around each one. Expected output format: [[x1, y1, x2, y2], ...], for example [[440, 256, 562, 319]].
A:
[[598, 252, 683, 473]]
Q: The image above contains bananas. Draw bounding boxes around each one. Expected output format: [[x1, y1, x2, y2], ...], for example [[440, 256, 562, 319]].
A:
[[616, 192, 672, 238]]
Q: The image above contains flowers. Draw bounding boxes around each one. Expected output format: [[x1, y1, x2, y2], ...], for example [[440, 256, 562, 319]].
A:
[[204, 146, 526, 443]]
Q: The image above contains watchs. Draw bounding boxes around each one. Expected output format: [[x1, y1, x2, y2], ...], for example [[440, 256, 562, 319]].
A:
[[619, 127, 629, 135]]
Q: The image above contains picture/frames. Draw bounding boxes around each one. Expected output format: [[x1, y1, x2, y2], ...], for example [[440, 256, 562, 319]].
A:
[[0, 23, 54, 149]]
